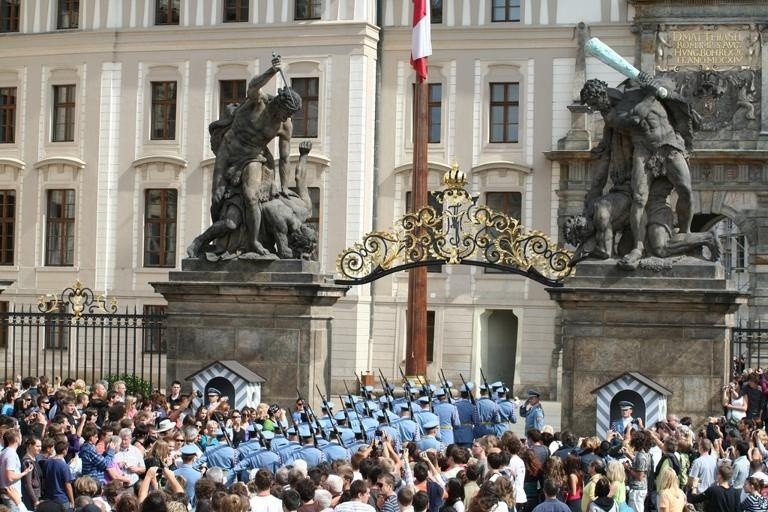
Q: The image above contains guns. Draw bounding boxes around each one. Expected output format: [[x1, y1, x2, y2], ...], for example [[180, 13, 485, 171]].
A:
[[216, 369, 492, 454]]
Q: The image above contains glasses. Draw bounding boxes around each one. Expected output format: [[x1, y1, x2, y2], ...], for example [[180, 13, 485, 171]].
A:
[[175, 439, 184, 442], [27, 398, 32, 401], [195, 424, 203, 429], [242, 414, 251, 418], [233, 415, 241, 419], [376, 481, 385, 487], [42, 401, 50, 404]]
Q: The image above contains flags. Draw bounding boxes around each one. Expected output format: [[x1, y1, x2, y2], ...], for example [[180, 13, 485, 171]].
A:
[[409, 0, 433, 86]]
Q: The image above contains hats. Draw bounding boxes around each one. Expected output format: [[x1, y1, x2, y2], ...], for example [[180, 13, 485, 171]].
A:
[[423, 421, 439, 429], [618, 401, 635, 410], [155, 419, 176, 432], [273, 420, 368, 439], [301, 381, 510, 421], [181, 445, 198, 456], [267, 404, 279, 415], [527, 389, 541, 398], [248, 424, 275, 440], [207, 387, 221, 396], [215, 428, 231, 438]]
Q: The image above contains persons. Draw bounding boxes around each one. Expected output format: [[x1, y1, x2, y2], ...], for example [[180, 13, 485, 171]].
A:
[[733, 353, 750, 379], [0, 369, 768, 512], [187, 52, 318, 255], [561, 69, 723, 266]]
